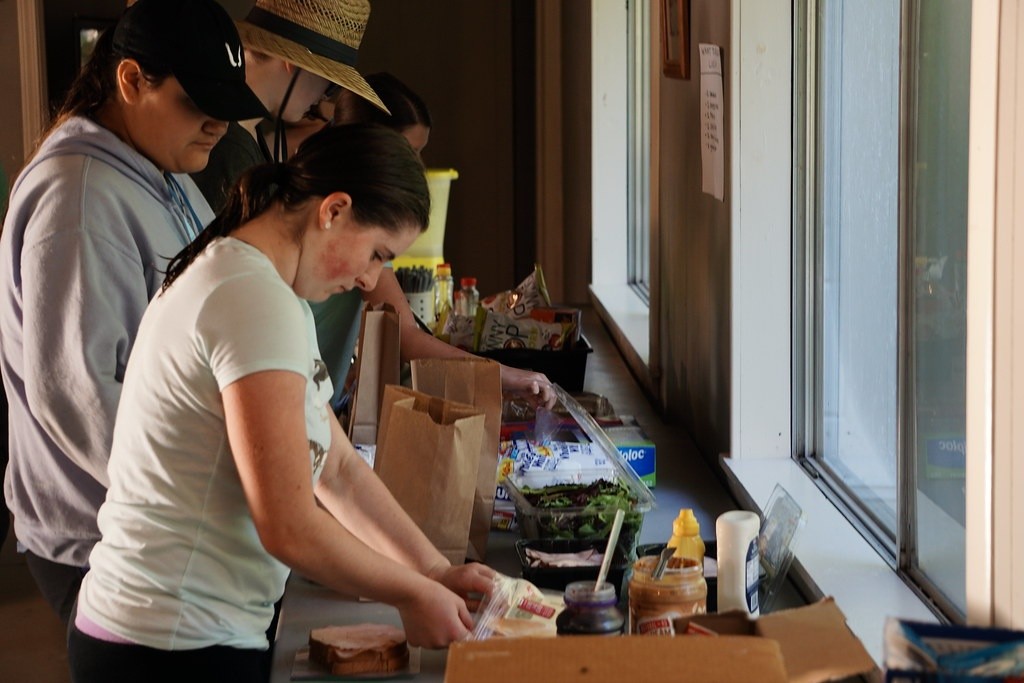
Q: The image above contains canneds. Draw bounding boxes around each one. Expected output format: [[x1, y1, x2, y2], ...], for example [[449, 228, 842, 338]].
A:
[[628, 555, 707, 639], [555, 580, 625, 637]]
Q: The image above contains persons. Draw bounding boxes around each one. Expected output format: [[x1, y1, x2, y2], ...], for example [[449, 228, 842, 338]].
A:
[[65, 120, 509, 683], [0, 2, 274, 624], [257, 60, 343, 162], [189, 0, 394, 216], [302, 71, 567, 421]]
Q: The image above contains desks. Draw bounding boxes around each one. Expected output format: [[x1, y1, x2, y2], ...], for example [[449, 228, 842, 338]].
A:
[[265, 303, 871, 683]]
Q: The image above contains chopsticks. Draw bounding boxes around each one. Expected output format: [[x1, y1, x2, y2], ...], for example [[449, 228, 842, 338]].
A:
[[393, 264, 436, 294]]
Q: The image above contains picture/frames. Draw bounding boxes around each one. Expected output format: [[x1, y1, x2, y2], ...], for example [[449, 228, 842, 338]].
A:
[[659, 0, 689, 78]]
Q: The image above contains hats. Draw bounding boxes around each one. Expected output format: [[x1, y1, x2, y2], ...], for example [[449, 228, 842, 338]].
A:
[[232, 0, 392, 116], [112, 0, 272, 122]]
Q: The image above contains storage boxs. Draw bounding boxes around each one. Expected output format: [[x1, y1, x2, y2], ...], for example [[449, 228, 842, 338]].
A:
[[391, 165, 458, 312], [501, 414, 657, 487], [882, 616, 1024, 682], [471, 333, 594, 392], [443, 596, 877, 683]]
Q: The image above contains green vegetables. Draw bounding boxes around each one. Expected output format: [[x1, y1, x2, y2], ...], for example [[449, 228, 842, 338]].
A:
[[514, 476, 642, 542]]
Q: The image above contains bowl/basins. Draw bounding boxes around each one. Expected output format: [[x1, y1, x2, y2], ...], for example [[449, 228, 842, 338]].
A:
[[502, 465, 652, 563]]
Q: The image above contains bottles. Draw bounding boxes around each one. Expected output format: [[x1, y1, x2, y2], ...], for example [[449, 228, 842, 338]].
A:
[[665, 508, 706, 582], [432, 264, 454, 322], [715, 509, 761, 621], [454, 276, 479, 317], [554, 582, 626, 638]]
